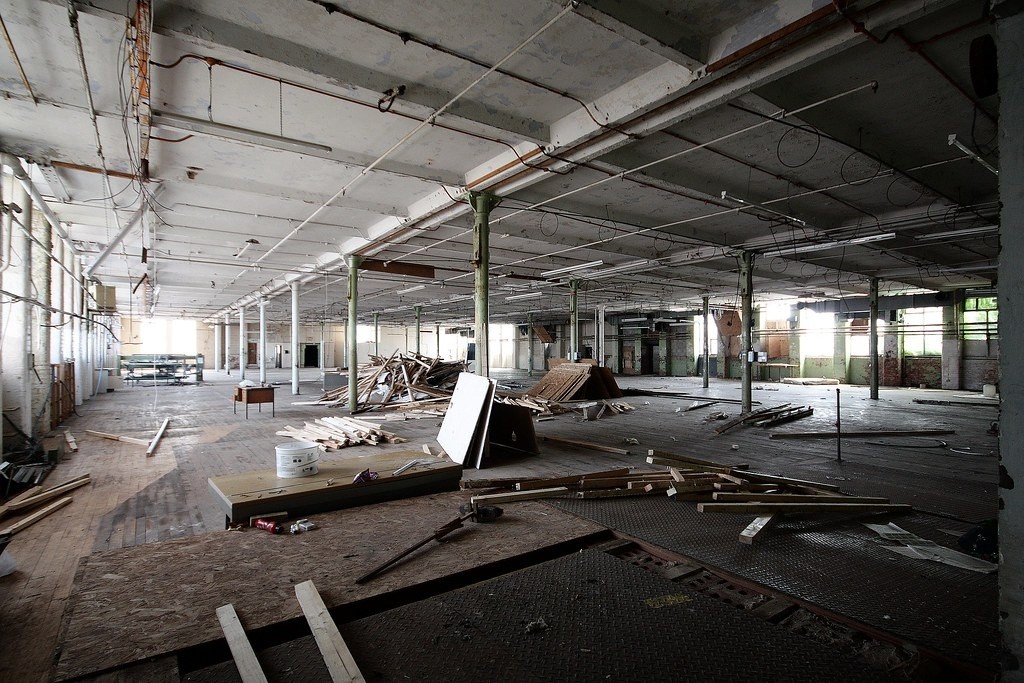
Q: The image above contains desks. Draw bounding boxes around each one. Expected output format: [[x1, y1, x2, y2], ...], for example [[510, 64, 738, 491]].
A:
[[233, 384, 280, 419]]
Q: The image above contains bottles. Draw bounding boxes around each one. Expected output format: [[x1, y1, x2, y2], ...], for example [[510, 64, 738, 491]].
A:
[[255, 519, 284, 534]]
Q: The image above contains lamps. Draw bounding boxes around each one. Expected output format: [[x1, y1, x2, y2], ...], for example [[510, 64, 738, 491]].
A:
[[914, 225, 1000, 242], [400, 259, 604, 303], [763, 232, 896, 259]]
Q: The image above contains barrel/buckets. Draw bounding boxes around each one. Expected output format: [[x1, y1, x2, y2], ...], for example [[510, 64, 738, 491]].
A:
[[275, 442, 320, 478], [983, 385, 996, 396], [758, 352, 767, 362]]
